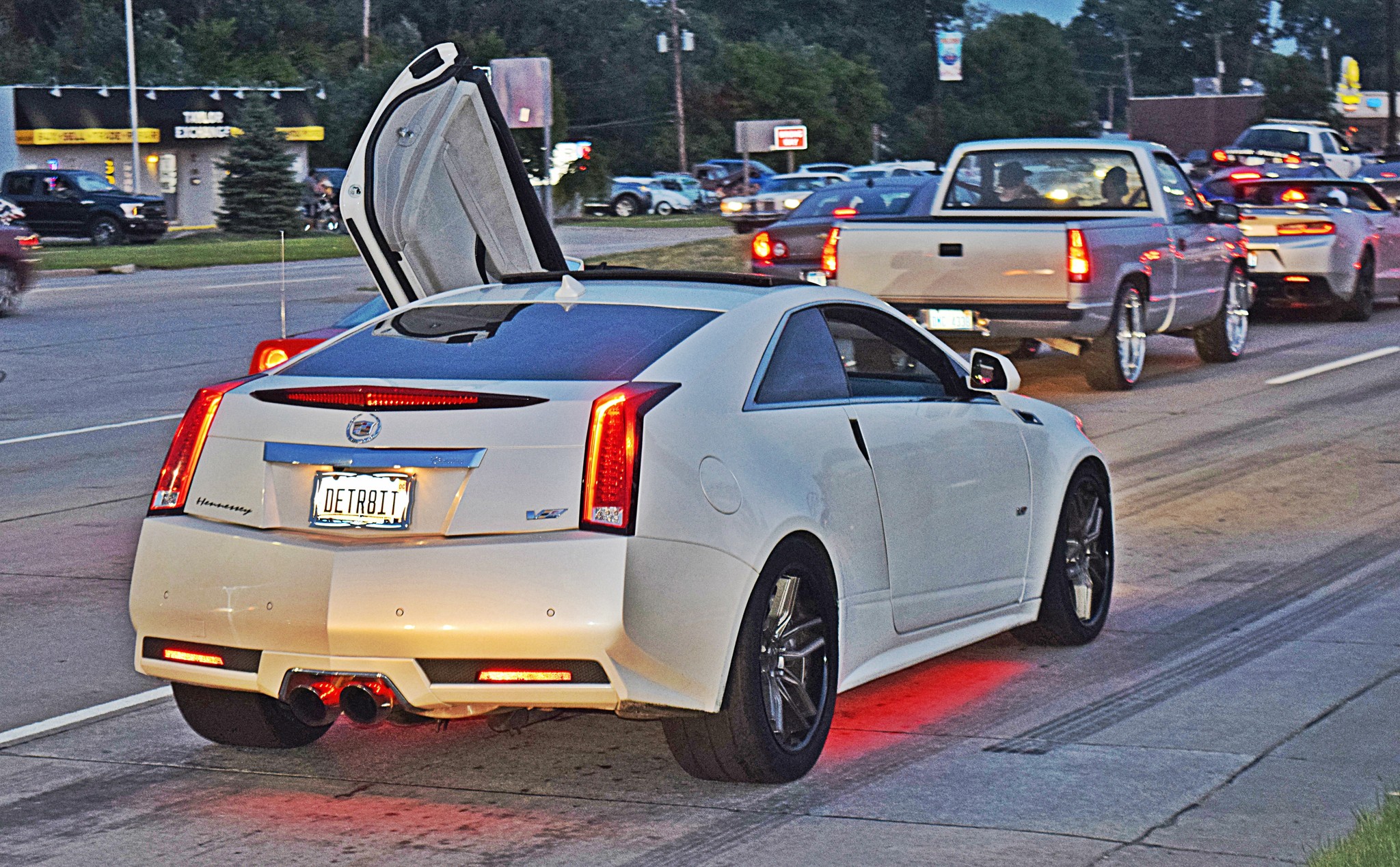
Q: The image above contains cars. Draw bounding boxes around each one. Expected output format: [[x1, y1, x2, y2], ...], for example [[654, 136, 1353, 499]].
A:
[[310, 166, 347, 211], [126, 38, 1119, 786], [580, 118, 1400, 322], [0, 197, 43, 321]]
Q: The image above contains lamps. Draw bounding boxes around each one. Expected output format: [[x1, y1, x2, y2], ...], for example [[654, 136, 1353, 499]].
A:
[[267, 80, 282, 99], [312, 81, 326, 100], [207, 80, 222, 100], [232, 81, 246, 99], [49, 77, 63, 97], [144, 79, 157, 100], [97, 77, 110, 97]]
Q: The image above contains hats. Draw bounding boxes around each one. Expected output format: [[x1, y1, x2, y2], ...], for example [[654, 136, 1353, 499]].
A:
[[999, 161, 1033, 181]]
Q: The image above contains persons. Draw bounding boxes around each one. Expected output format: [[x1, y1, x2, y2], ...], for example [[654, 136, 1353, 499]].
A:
[[302, 170, 324, 231], [997, 161, 1033, 202], [1100, 166, 1129, 206]]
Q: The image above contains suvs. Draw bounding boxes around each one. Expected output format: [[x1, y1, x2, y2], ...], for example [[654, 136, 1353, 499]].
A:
[[0, 168, 171, 247]]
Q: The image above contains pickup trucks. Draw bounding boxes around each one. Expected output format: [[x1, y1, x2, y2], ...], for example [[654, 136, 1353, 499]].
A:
[[830, 134, 1252, 392]]
